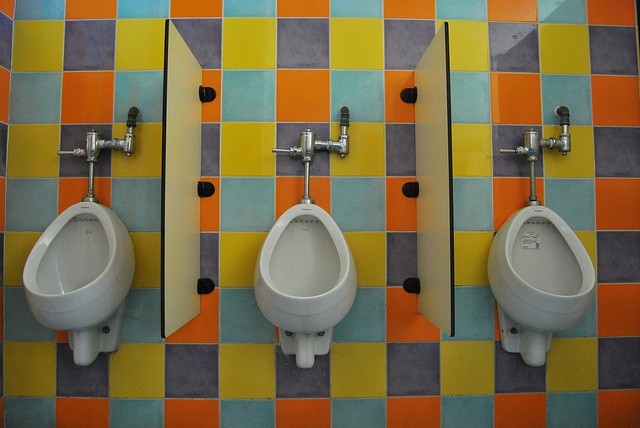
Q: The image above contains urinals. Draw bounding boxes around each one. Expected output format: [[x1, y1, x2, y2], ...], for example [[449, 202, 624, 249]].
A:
[[253, 203, 357, 370], [21, 200, 136, 366], [488, 204, 597, 369]]
